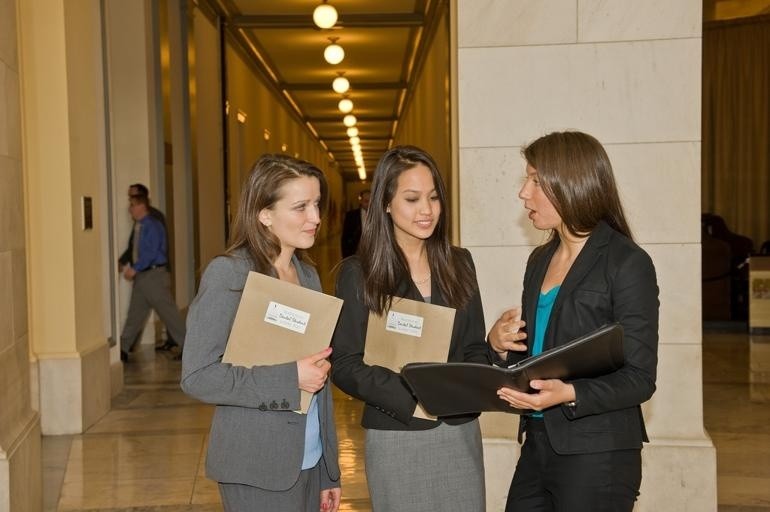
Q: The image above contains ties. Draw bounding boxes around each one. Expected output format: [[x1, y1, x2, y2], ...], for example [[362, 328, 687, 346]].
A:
[[132, 222, 141, 265]]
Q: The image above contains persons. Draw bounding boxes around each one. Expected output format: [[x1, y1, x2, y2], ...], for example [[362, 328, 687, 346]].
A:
[[326, 144, 491, 511], [177, 153, 342, 511], [118, 184, 178, 354], [120, 194, 186, 363], [340, 189, 372, 259], [485, 130, 661, 512]]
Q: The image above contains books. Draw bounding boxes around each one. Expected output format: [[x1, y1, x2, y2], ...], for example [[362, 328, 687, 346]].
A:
[[363, 293, 457, 421], [222, 272, 346, 415], [401, 321, 624, 415]]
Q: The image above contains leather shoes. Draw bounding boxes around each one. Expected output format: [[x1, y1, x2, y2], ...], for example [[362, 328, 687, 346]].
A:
[[154, 339, 183, 361], [120, 349, 129, 363]]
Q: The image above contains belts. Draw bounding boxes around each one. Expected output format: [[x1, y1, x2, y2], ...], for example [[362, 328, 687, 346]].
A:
[[142, 263, 166, 272]]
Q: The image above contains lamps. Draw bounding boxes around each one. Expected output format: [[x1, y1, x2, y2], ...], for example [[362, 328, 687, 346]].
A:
[[310, 1, 372, 186]]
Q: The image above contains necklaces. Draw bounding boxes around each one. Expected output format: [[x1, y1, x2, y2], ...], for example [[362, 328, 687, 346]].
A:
[[414, 275, 431, 284]]
